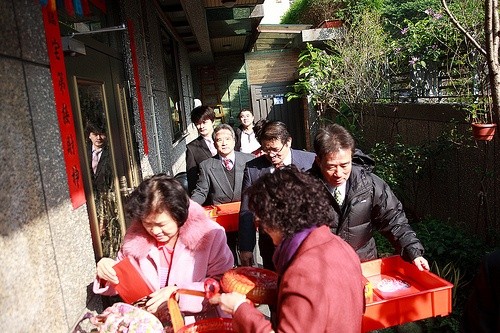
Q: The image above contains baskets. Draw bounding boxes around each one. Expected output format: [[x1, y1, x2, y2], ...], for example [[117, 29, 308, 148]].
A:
[[176, 318, 236, 333], [219, 266, 279, 305]]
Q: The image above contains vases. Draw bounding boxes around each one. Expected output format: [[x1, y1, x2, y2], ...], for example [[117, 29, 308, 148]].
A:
[[471, 123, 496, 141]]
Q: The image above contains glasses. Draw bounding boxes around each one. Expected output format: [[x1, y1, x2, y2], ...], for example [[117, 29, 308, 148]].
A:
[[260, 142, 287, 155]]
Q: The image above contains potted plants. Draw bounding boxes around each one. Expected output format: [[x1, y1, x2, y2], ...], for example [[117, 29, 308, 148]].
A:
[[308, 0, 342, 28]]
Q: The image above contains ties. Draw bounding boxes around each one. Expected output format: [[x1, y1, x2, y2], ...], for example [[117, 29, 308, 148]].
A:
[[91, 148, 102, 175], [244, 131, 254, 145], [334, 188, 344, 205], [223, 158, 230, 171], [207, 138, 215, 154]]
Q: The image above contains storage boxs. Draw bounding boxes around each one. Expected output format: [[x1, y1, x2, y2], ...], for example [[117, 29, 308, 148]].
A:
[[202, 201, 241, 231], [360, 255, 453, 333]]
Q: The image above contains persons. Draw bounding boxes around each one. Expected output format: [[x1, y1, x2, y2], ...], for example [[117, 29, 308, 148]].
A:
[[185, 105, 317, 311], [93, 173, 234, 314], [87, 122, 112, 184], [305, 124, 430, 271], [209, 164, 366, 333]]
